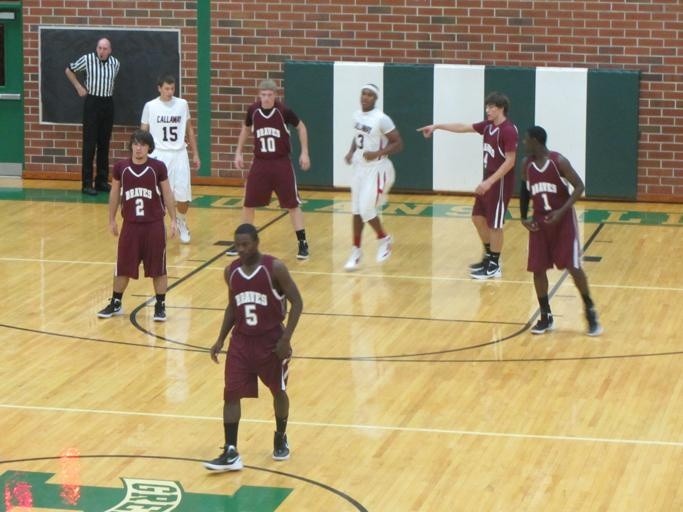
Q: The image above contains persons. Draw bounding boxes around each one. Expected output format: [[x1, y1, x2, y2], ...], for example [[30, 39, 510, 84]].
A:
[[417, 90, 519, 279], [225, 80, 310, 259], [203, 224, 304, 473], [65, 38, 120, 194], [141, 74, 202, 243], [522, 125, 602, 336], [343, 84, 402, 273], [96, 130, 177, 321]]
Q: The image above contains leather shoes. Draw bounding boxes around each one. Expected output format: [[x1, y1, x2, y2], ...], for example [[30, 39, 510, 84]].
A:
[[82, 188, 99, 197], [95, 183, 111, 192]]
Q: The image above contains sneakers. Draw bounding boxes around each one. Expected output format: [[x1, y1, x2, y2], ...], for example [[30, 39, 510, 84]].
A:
[[531, 312, 554, 335], [176, 220, 190, 243], [468, 253, 502, 280], [204, 444, 243, 472], [154, 303, 168, 322], [374, 237, 394, 261], [346, 248, 363, 268], [586, 308, 601, 337], [272, 430, 291, 461], [296, 242, 309, 259], [96, 298, 124, 319]]
